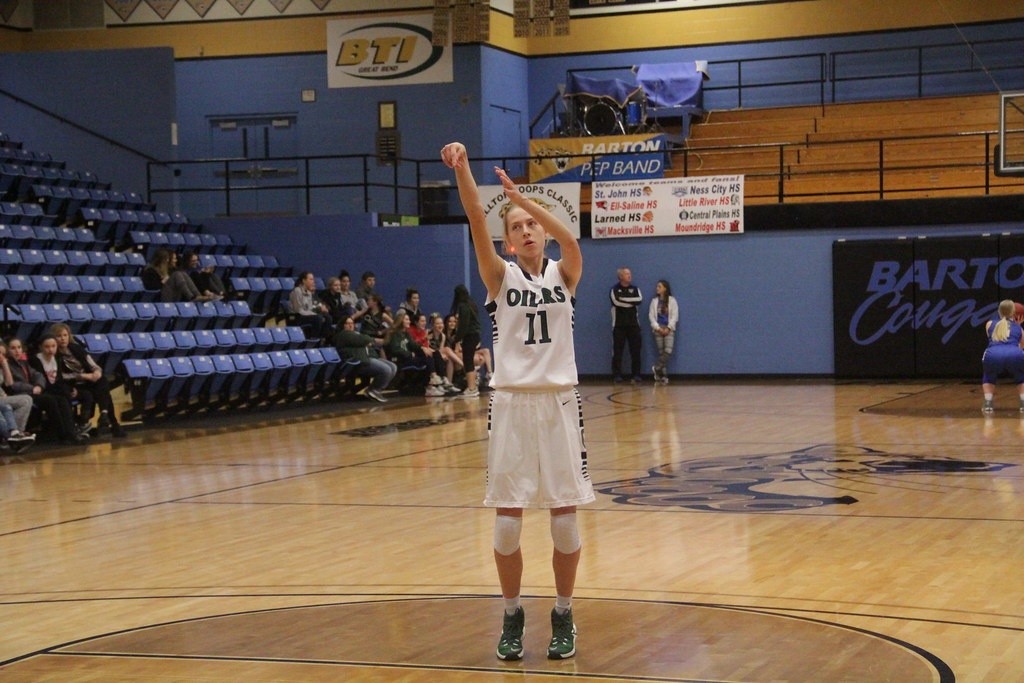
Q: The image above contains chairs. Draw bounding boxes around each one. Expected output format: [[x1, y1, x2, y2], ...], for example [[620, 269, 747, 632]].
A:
[[2, 131, 344, 450]]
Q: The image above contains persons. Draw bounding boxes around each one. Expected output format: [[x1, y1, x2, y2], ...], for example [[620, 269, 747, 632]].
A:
[[142, 249, 225, 301], [981, 300, 1024, 412], [610, 267, 644, 384], [649, 280, 678, 383], [0, 323, 128, 452], [286, 271, 493, 402], [441, 142, 595, 661]]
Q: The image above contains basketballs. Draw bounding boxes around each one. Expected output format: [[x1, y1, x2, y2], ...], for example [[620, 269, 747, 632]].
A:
[[1012, 302, 1024, 323]]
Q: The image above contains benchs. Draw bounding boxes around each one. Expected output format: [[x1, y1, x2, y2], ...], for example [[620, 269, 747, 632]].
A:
[[510, 91, 1024, 205]]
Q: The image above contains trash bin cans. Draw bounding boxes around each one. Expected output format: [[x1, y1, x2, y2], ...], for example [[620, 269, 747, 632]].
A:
[[419, 180, 452, 225]]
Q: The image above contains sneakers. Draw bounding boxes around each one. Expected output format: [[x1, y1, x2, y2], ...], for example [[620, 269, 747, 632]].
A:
[[651, 366, 670, 386], [610, 376, 624, 383], [629, 377, 642, 386], [547, 607, 577, 660], [367, 388, 391, 403], [496, 605, 526, 661]]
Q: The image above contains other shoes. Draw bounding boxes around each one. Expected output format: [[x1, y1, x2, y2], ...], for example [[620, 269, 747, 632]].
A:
[[5, 424, 130, 454], [425, 373, 494, 398]]
